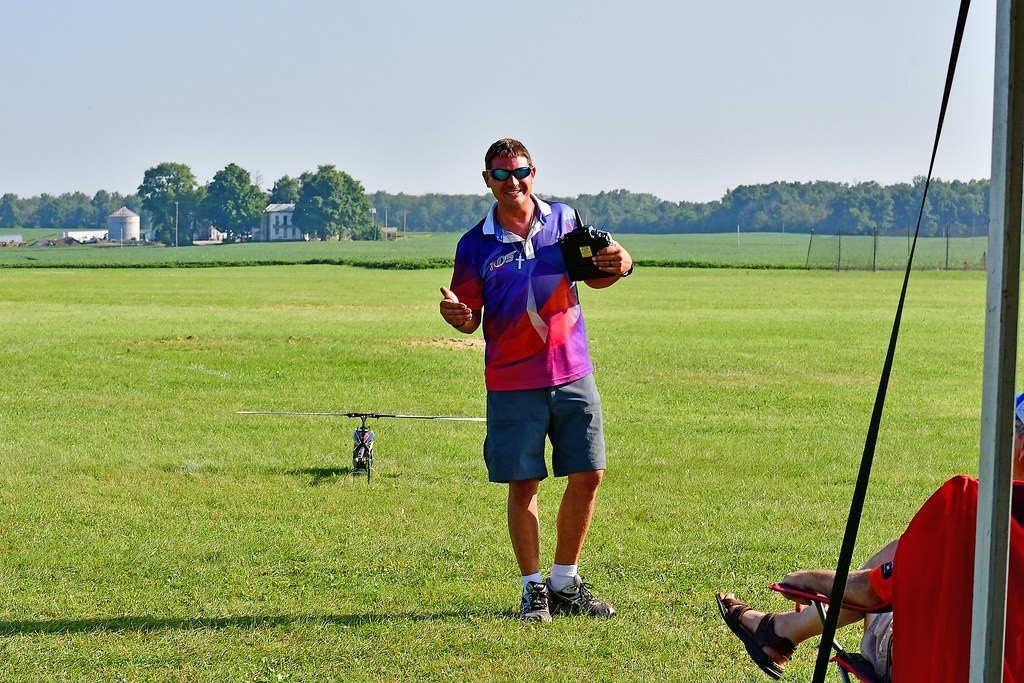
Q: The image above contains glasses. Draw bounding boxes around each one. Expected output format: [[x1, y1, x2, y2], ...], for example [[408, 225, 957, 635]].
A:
[[487, 167, 531, 181]]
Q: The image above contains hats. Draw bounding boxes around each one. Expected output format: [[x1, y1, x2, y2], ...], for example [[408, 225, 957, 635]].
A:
[[1015, 393, 1024, 430]]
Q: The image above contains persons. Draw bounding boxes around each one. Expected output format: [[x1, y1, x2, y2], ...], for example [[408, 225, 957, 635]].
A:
[[713, 388, 1024, 683], [439, 138, 633, 623]]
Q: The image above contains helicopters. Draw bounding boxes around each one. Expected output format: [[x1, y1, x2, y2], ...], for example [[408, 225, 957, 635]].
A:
[[238, 409, 489, 479]]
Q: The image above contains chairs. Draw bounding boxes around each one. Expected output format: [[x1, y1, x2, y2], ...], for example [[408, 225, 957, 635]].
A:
[[768, 473, 1024, 683]]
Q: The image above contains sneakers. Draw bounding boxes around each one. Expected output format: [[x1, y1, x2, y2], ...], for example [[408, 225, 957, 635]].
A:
[[547, 575, 617, 616], [519, 581, 552, 622]]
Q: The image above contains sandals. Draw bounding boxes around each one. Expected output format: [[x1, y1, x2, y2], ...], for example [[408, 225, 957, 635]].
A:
[[715, 591, 798, 680]]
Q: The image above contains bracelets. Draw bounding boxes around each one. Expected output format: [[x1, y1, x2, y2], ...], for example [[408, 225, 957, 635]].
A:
[[618, 264, 633, 277], [452, 322, 465, 328]]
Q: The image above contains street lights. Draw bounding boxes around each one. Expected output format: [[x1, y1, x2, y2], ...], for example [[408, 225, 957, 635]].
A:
[[175, 201, 180, 247]]
